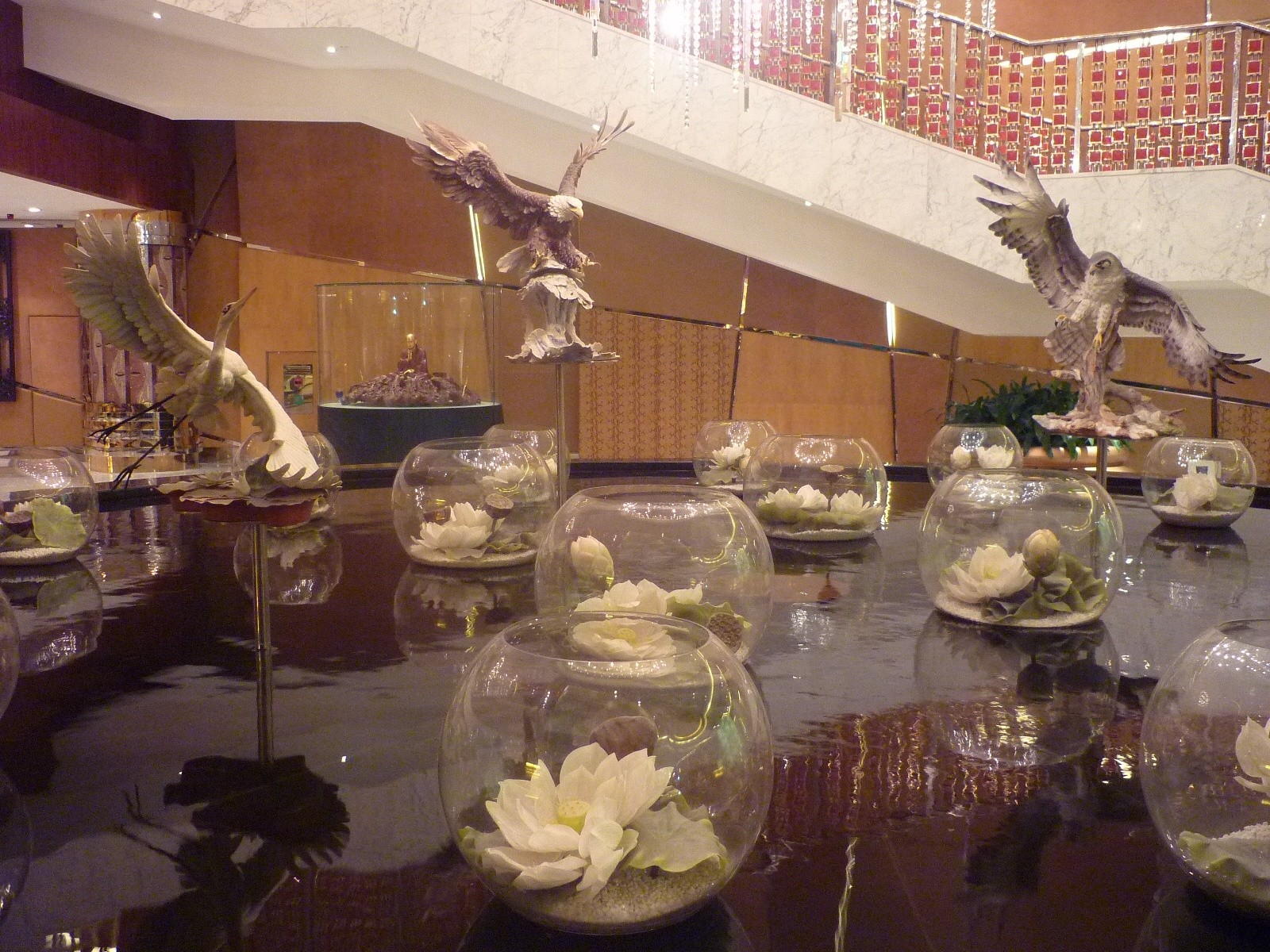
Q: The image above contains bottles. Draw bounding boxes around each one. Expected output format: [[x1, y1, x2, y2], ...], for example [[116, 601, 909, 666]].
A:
[[233, 518, 344, 605], [915, 467, 1124, 628], [925, 424, 1023, 490], [535, 482, 774, 685], [0, 446, 100, 565], [691, 420, 784, 496], [1134, 522, 1252, 609], [914, 607, 1121, 768], [743, 432, 888, 540], [1136, 617, 1270, 917], [0, 558, 105, 678], [435, 610, 776, 932], [389, 434, 556, 569], [1139, 435, 1258, 529]]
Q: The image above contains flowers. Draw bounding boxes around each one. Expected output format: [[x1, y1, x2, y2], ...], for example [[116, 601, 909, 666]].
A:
[[0, 445, 1269, 952]]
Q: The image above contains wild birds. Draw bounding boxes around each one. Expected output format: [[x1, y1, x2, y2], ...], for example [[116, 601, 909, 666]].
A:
[[64, 213, 341, 489]]
